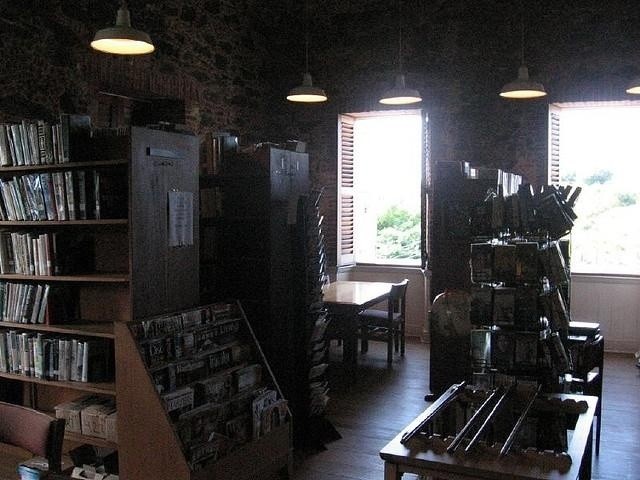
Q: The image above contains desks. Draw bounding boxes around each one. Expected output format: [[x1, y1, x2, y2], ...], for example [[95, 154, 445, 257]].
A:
[[322, 280, 400, 375]]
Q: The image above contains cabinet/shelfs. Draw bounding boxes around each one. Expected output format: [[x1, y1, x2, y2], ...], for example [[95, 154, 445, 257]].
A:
[[424, 160, 570, 403], [1, 127, 341, 479]]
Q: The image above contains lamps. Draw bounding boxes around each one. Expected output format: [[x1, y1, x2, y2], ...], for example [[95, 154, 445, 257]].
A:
[[499, 18, 548, 99], [377, 17, 424, 106], [90, 1, 156, 57], [286, 20, 328, 105]]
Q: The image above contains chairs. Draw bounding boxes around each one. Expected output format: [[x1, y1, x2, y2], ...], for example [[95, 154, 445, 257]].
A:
[[569, 334, 604, 458], [352, 279, 410, 365]]
